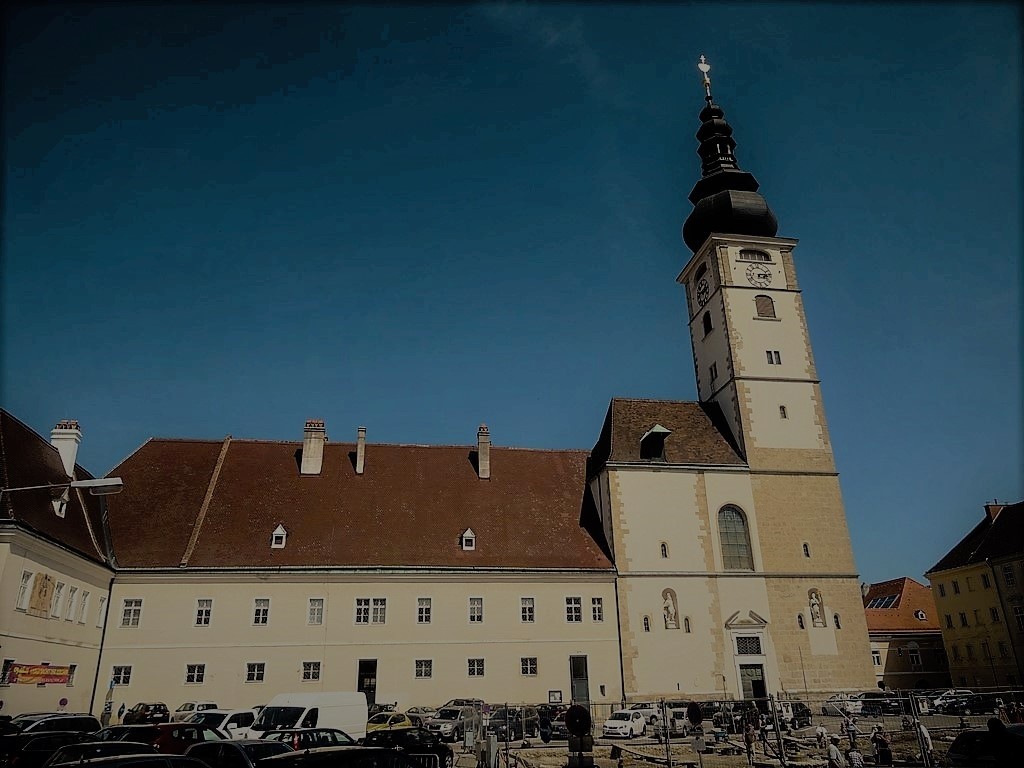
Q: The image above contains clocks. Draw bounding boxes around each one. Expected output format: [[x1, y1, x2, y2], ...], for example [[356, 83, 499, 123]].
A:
[[746, 262, 772, 288], [696, 279, 708, 306]]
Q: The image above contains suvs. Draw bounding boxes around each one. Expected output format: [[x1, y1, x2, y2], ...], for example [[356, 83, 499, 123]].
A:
[[123, 701, 171, 725], [93, 722, 230, 751], [172, 709, 260, 741], [254, 737, 427, 768], [170, 701, 218, 723], [257, 728, 363, 748], [364, 726, 455, 768]]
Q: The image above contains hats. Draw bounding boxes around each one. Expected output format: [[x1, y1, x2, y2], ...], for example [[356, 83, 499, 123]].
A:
[[872, 725, 877, 728], [831, 734, 841, 739], [912, 719, 920, 723], [875, 723, 883, 727]]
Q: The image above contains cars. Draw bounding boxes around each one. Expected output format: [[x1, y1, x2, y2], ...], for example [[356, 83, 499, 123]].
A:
[[482, 707, 541, 741], [855, 691, 902, 717], [0, 711, 210, 768], [898, 689, 984, 716], [602, 709, 647, 739], [549, 711, 595, 738], [404, 706, 441, 725], [185, 737, 294, 768], [366, 712, 412, 733], [628, 702, 663, 725], [425, 706, 479, 743], [653, 708, 694, 738], [534, 703, 568, 730], [820, 693, 864, 715], [698, 699, 812, 732]]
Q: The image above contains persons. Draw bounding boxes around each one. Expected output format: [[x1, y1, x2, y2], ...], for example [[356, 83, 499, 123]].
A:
[[759, 714, 774, 756], [181, 729, 197, 744], [744, 724, 756, 766], [901, 716, 910, 731], [996, 692, 1024, 723], [782, 723, 807, 750], [722, 707, 772, 733], [390, 702, 398, 712], [967, 718, 1024, 768], [491, 705, 497, 711], [664, 593, 676, 622], [146, 705, 166, 711], [912, 720, 934, 768], [816, 712, 895, 768], [809, 591, 822, 627]]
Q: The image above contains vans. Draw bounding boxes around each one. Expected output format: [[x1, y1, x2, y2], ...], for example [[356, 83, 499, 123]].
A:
[[243, 691, 369, 744]]
